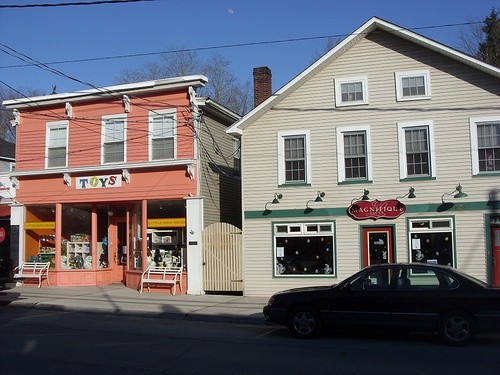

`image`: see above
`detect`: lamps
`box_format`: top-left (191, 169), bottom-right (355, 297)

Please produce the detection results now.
top-left (454, 182), bottom-right (467, 198)
top-left (159, 202), bottom-right (163, 210)
top-left (363, 189), bottom-right (369, 200)
top-left (51, 207), bottom-right (55, 213)
top-left (407, 186), bottom-right (417, 198)
top-left (272, 192), bottom-right (283, 204)
top-left (315, 191), bottom-right (326, 202)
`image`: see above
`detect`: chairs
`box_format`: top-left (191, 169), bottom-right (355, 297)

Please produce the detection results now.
top-left (401, 271), bottom-right (411, 288)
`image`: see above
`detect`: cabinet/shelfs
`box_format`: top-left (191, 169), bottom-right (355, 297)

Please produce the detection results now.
top-left (147, 228), bottom-right (185, 267)
top-left (67, 241), bottom-right (104, 269)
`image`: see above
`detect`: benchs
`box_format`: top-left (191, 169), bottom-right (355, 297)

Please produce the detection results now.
top-left (139, 266), bottom-right (183, 296)
top-left (12, 262), bottom-right (51, 288)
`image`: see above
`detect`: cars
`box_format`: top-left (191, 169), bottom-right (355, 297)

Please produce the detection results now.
top-left (263, 263), bottom-right (500, 339)
